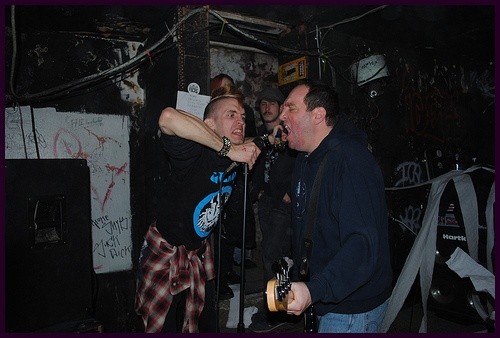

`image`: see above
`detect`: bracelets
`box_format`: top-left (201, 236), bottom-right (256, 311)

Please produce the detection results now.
top-left (263, 133), bottom-right (273, 149)
top-left (217, 136), bottom-right (231, 157)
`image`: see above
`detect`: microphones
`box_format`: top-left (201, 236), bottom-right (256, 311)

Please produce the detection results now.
top-left (222, 137), bottom-right (263, 180)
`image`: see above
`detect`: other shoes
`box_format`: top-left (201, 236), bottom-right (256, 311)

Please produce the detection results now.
top-left (218, 285), bottom-right (234, 300)
top-left (248, 309), bottom-right (289, 332)
top-left (221, 265), bottom-right (242, 285)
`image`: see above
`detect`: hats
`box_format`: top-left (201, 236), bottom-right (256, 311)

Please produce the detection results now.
top-left (258, 86), bottom-right (285, 103)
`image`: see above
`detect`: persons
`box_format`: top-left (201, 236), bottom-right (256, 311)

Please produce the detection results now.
top-left (134, 94), bottom-right (287, 332)
top-left (211, 73), bottom-right (303, 299)
top-left (279, 79), bottom-right (391, 332)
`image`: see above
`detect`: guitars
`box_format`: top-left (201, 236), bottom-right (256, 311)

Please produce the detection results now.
top-left (266, 266), bottom-right (319, 333)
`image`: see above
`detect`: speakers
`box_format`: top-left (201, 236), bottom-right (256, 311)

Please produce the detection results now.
top-left (5, 159), bottom-right (93, 333)
